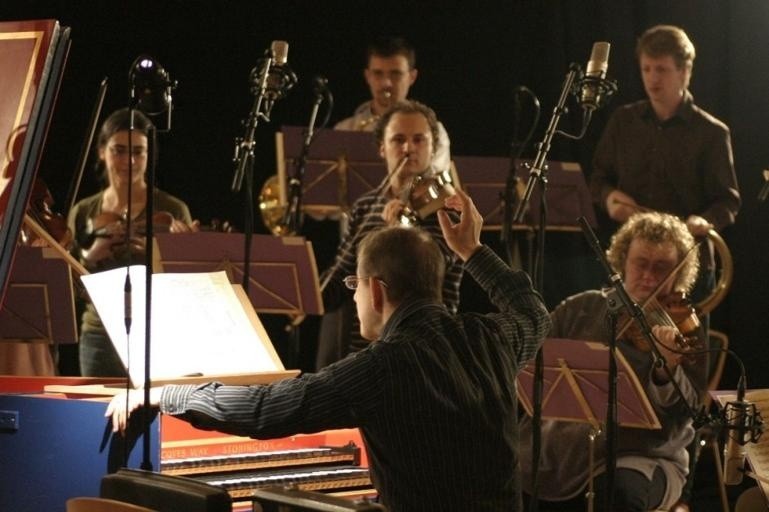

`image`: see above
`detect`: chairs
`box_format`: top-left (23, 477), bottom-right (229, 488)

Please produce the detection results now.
top-left (674, 326), bottom-right (731, 512)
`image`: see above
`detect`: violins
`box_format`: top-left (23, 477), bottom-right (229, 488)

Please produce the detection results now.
top-left (395, 171), bottom-right (452, 232)
top-left (85, 214), bottom-right (230, 255)
top-left (18, 176), bottom-right (92, 303)
top-left (620, 294), bottom-right (704, 366)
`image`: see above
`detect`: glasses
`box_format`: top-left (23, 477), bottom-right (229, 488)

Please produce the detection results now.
top-left (344, 275), bottom-right (390, 291)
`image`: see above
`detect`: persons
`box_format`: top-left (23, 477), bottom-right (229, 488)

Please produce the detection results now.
top-left (588, 25), bottom-right (742, 312)
top-left (68, 109), bottom-right (191, 377)
top-left (333, 38), bottom-right (452, 179)
top-left (523, 213), bottom-right (704, 509)
top-left (105, 178), bottom-right (552, 511)
top-left (289, 101), bottom-right (470, 354)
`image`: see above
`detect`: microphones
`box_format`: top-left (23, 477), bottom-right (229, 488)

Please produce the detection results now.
top-left (263, 40), bottom-right (288, 115)
top-left (725, 400), bottom-right (752, 485)
top-left (580, 41), bottom-right (611, 107)
